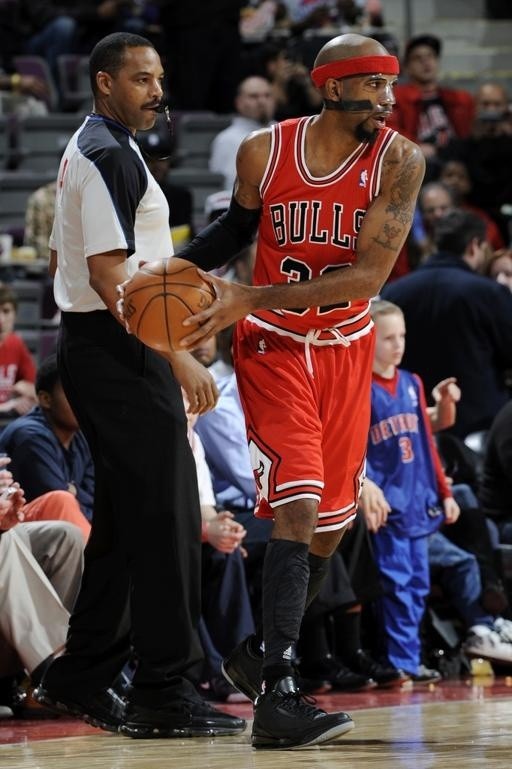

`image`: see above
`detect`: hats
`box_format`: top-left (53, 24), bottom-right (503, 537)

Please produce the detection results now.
top-left (402, 32), bottom-right (444, 66)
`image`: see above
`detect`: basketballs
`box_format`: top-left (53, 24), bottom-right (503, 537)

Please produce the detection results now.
top-left (121, 256), bottom-right (217, 351)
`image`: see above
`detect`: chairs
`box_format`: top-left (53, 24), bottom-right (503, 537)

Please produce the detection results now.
top-left (1, 45), bottom-right (251, 393)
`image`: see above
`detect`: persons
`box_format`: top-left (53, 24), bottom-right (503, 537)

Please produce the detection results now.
top-left (0, 1), bottom-right (512, 716)
top-left (116, 34), bottom-right (426, 749)
top-left (49, 32), bottom-right (248, 738)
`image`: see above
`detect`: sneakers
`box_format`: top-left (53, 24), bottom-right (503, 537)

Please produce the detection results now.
top-left (402, 663), bottom-right (443, 684)
top-left (247, 689), bottom-right (356, 751)
top-left (114, 688), bottom-right (247, 740)
top-left (466, 614), bottom-right (511, 666)
top-left (295, 663), bottom-right (332, 692)
top-left (342, 649), bottom-right (405, 685)
top-left (31, 652), bottom-right (127, 734)
top-left (319, 653), bottom-right (380, 693)
top-left (220, 633), bottom-right (267, 703)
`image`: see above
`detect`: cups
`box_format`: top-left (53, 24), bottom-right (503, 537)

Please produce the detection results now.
top-left (2, 234), bottom-right (12, 262)
top-left (1, 453), bottom-right (10, 495)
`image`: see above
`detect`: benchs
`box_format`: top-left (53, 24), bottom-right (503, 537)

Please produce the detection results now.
top-left (368, 1), bottom-right (512, 110)
